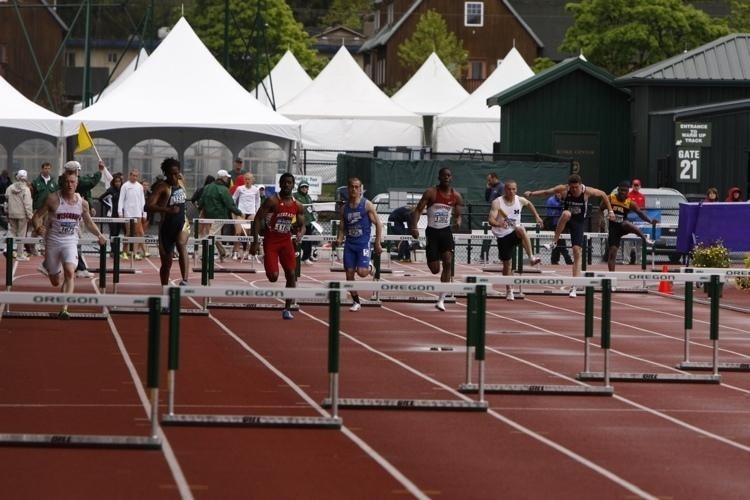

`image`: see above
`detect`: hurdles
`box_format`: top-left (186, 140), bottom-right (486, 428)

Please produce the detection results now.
top-left (70, 217), bottom-right (700, 274)
top-left (0, 291), bottom-right (170, 447)
top-left (374, 235), bottom-right (456, 302)
top-left (110, 235), bottom-right (213, 316)
top-left (291, 235), bottom-right (386, 307)
top-left (318, 280), bottom-right (493, 411)
top-left (204, 234), bottom-right (299, 311)
top-left (575, 270), bottom-right (725, 383)
top-left (453, 233), bottom-right (527, 299)
top-left (457, 275), bottom-right (615, 396)
top-left (1, 236), bottom-right (112, 318)
top-left (583, 231), bottom-right (649, 293)
top-left (511, 232), bottom-right (587, 295)
top-left (160, 285), bottom-right (346, 427)
top-left (675, 266), bottom-right (749, 371)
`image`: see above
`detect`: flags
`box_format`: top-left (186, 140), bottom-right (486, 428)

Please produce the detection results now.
top-left (72, 123), bottom-right (92, 155)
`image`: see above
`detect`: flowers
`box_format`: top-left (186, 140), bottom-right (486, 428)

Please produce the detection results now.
top-left (692, 238), bottom-right (731, 268)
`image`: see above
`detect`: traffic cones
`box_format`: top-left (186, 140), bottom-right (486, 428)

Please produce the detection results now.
top-left (656, 263), bottom-right (675, 295)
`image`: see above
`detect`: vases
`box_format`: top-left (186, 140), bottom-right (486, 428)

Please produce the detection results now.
top-left (704, 282), bottom-right (724, 298)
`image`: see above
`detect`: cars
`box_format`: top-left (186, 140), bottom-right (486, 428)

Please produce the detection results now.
top-left (370, 193), bottom-right (428, 247)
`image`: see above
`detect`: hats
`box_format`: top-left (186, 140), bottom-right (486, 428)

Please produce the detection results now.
top-left (17, 169), bottom-right (28, 179)
top-left (218, 169), bottom-right (232, 179)
top-left (65, 161), bottom-right (82, 170)
top-left (633, 179), bottom-right (641, 186)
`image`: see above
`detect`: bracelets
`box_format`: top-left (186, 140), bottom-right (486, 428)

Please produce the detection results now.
top-left (608, 211), bottom-right (613, 213)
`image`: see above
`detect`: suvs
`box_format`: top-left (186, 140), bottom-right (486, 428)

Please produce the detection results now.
top-left (596, 182), bottom-right (692, 263)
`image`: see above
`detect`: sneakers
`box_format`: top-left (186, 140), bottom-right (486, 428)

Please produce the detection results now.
top-left (569, 286), bottom-right (578, 298)
top-left (349, 301), bottom-right (362, 313)
top-left (434, 298), bottom-right (446, 311)
top-left (36, 263), bottom-right (49, 276)
top-left (504, 288), bottom-right (514, 301)
top-left (641, 238), bottom-right (657, 247)
top-left (543, 241), bottom-right (558, 251)
top-left (57, 309), bottom-right (72, 319)
top-left (529, 255), bottom-right (541, 267)
top-left (282, 309), bottom-right (294, 320)
top-left (16, 254), bottom-right (32, 262)
top-left (76, 270), bottom-right (97, 279)
top-left (111, 250), bottom-right (319, 264)
top-left (368, 263), bottom-right (377, 276)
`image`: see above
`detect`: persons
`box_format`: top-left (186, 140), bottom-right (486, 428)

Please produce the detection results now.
top-left (546, 184), bottom-right (574, 264)
top-left (723, 187), bottom-right (744, 201)
top-left (1, 155), bottom-right (384, 319)
top-left (484, 172), bottom-right (505, 206)
top-left (387, 205), bottom-right (423, 262)
top-left (522, 173), bottom-right (617, 298)
top-left (629, 179), bottom-right (645, 209)
top-left (599, 180), bottom-right (661, 270)
top-left (410, 168), bottom-right (463, 312)
top-left (487, 179), bottom-right (544, 300)
top-left (702, 186), bottom-right (720, 202)
